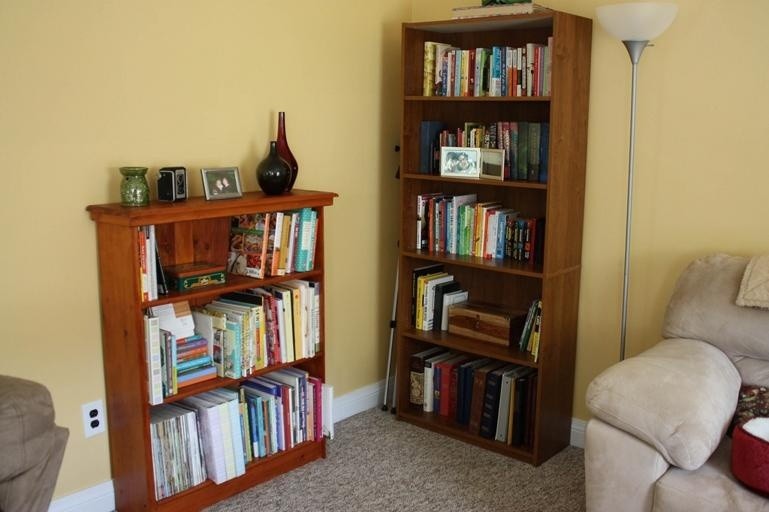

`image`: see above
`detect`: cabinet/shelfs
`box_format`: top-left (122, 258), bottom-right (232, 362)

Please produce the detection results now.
top-left (390, 11), bottom-right (595, 468)
top-left (84, 187), bottom-right (340, 511)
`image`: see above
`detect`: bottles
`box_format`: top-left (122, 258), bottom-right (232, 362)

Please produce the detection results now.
top-left (119, 167), bottom-right (151, 206)
top-left (254, 111), bottom-right (298, 195)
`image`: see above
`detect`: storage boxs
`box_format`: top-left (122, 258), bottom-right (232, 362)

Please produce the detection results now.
top-left (446, 297), bottom-right (527, 349)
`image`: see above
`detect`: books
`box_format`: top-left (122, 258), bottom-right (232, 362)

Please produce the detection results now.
top-left (227, 205), bottom-right (320, 279)
top-left (136, 223), bottom-right (226, 303)
top-left (415, 195), bottom-right (545, 267)
top-left (411, 264), bottom-right (543, 364)
top-left (145, 282), bottom-right (336, 501)
top-left (419, 120), bottom-right (548, 184)
top-left (410, 348), bottom-right (534, 445)
top-left (423, 36), bottom-right (554, 97)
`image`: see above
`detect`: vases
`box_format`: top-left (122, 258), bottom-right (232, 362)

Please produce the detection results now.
top-left (256, 111), bottom-right (299, 196)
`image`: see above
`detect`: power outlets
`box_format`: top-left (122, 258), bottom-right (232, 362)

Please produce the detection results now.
top-left (81, 399), bottom-right (108, 439)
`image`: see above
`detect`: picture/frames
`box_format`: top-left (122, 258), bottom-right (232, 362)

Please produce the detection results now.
top-left (439, 146), bottom-right (506, 182)
top-left (199, 166), bottom-right (242, 201)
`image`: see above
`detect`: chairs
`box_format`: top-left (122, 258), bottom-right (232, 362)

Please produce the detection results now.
top-left (583, 253), bottom-right (766, 510)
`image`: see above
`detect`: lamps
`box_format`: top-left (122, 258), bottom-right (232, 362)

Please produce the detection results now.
top-left (595, 5), bottom-right (680, 364)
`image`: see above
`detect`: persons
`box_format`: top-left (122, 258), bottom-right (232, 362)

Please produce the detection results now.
top-left (213, 178), bottom-right (224, 196)
top-left (222, 176), bottom-right (234, 194)
